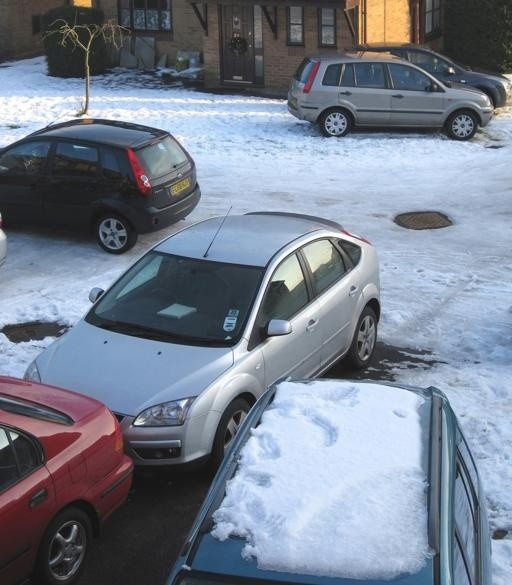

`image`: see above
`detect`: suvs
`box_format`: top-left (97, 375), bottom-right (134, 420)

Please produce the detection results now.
top-left (358, 42), bottom-right (509, 107)
top-left (291, 55), bottom-right (493, 142)
top-left (1, 117), bottom-right (202, 256)
top-left (165, 375), bottom-right (491, 585)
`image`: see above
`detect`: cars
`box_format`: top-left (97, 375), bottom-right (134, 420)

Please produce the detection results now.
top-left (0, 374), bottom-right (126, 585)
top-left (23, 206), bottom-right (381, 466)
